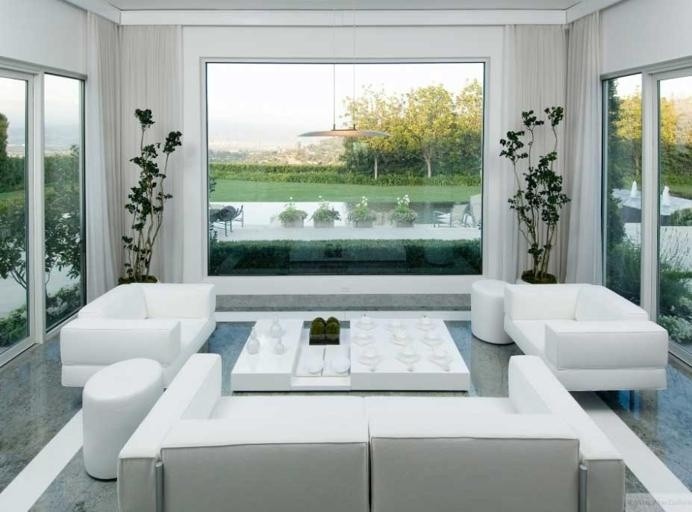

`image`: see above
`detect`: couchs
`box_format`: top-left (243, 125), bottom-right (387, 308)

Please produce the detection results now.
top-left (501, 281), bottom-right (669, 408)
top-left (116, 351), bottom-right (630, 511)
top-left (58, 282), bottom-right (221, 388)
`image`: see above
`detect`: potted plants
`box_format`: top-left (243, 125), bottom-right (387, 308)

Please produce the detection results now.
top-left (500, 105), bottom-right (573, 282)
top-left (117, 107), bottom-right (186, 285)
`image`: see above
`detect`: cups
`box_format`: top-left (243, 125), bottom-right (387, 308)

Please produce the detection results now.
top-left (351, 311), bottom-right (456, 372)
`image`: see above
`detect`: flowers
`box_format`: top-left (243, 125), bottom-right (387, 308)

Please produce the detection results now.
top-left (347, 196), bottom-right (377, 221)
top-left (278, 196), bottom-right (306, 220)
top-left (390, 194), bottom-right (419, 223)
top-left (313, 195), bottom-right (340, 222)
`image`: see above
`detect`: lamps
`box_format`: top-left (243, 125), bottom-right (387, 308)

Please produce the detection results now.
top-left (299, 9), bottom-right (390, 137)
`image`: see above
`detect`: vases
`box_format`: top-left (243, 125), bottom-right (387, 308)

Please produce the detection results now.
top-left (282, 219), bottom-right (304, 226)
top-left (396, 219), bottom-right (415, 227)
top-left (354, 219), bottom-right (373, 229)
top-left (314, 220), bottom-right (334, 228)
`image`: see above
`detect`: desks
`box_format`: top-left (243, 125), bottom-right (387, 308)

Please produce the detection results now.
top-left (79, 361), bottom-right (168, 482)
top-left (471, 279), bottom-right (519, 346)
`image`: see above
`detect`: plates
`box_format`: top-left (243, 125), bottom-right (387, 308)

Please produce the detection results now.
top-left (304, 354), bottom-right (324, 374)
top-left (331, 356), bottom-right (350, 373)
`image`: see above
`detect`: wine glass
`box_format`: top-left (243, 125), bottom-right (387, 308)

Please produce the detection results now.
top-left (245, 328), bottom-right (260, 354)
top-left (272, 316), bottom-right (281, 337)
top-left (275, 331), bottom-right (284, 355)
